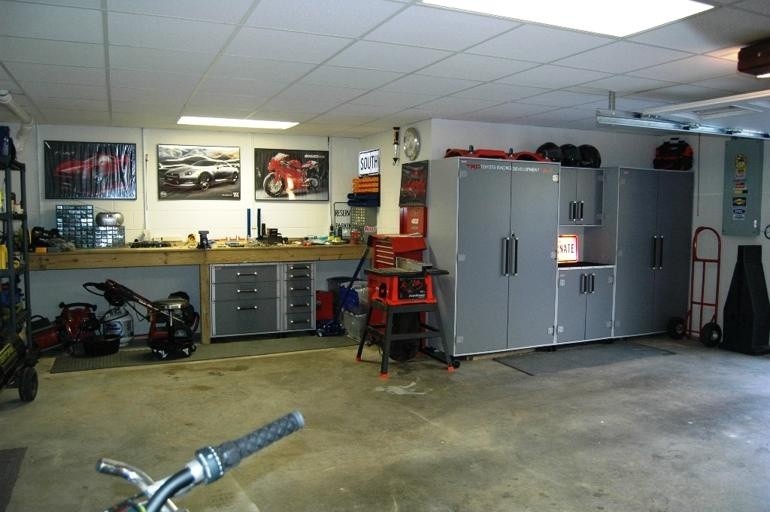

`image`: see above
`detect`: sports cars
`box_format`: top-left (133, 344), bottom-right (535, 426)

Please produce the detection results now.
top-left (54, 154), bottom-right (129, 185)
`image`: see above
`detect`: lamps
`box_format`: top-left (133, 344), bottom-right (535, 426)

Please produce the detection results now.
top-left (737, 38), bottom-right (769, 79)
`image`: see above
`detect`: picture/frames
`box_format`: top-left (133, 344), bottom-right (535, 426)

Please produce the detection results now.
top-left (358, 148), bottom-right (380, 178)
top-left (44, 140), bottom-right (330, 201)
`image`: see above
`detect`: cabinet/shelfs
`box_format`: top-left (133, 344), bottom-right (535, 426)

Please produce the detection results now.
top-left (209, 261), bottom-right (317, 339)
top-left (558, 167), bottom-right (604, 226)
top-left (1, 158), bottom-right (38, 402)
top-left (427, 160), bottom-right (557, 357)
top-left (557, 260), bottom-right (615, 346)
top-left (585, 167), bottom-right (693, 346)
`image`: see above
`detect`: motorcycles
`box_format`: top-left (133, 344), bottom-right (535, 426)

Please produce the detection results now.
top-left (263, 153), bottom-right (319, 197)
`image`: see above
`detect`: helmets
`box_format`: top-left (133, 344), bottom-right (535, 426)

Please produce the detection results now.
top-left (537, 142), bottom-right (601, 168)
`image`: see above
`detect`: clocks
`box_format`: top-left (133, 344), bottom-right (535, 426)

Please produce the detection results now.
top-left (403, 128), bottom-right (420, 161)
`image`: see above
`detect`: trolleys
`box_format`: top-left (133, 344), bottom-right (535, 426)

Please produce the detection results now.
top-left (666, 225), bottom-right (724, 349)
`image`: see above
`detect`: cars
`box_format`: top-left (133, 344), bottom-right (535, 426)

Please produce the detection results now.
top-left (164, 159), bottom-right (238, 191)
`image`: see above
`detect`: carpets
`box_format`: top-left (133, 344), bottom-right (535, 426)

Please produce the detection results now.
top-left (0, 446), bottom-right (29, 512)
top-left (493, 341), bottom-right (676, 376)
top-left (49, 334), bottom-right (360, 374)
